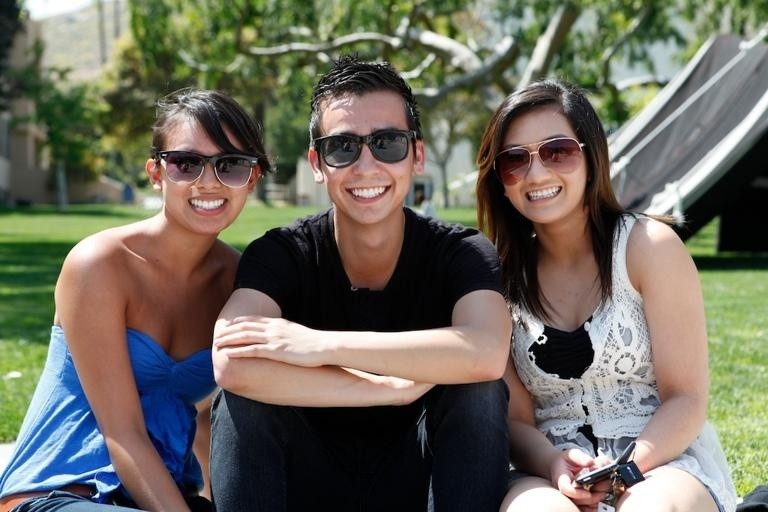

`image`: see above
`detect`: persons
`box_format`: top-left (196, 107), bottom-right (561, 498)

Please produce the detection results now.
top-left (0, 87), bottom-right (275, 511)
top-left (474, 79), bottom-right (738, 512)
top-left (208, 51), bottom-right (514, 512)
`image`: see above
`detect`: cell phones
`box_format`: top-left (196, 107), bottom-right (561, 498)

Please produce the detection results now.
top-left (575, 463), bottom-right (617, 485)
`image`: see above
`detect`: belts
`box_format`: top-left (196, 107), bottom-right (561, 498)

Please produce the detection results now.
top-left (0, 484), bottom-right (96, 512)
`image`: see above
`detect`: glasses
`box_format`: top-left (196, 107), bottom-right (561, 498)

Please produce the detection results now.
top-left (493, 135), bottom-right (586, 186)
top-left (154, 150), bottom-right (259, 189)
top-left (310, 129), bottom-right (416, 169)
